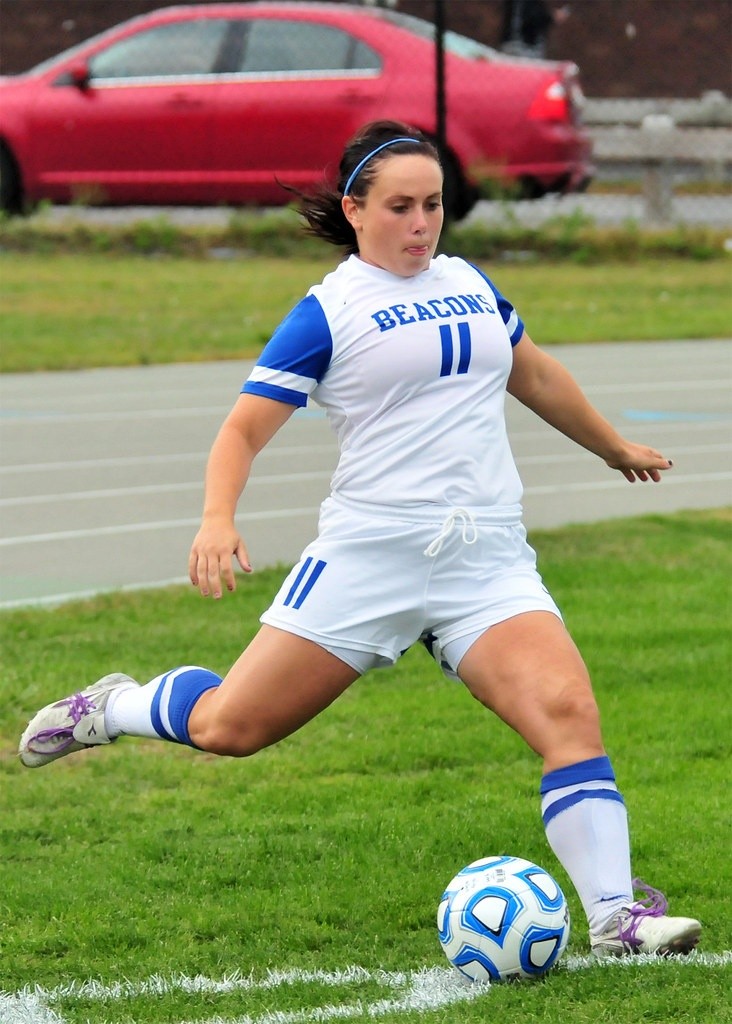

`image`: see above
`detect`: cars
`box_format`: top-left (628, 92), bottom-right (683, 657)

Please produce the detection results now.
top-left (0, 1), bottom-right (596, 223)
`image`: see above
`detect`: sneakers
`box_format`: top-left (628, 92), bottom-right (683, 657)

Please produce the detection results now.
top-left (19, 671), bottom-right (141, 769)
top-left (587, 901), bottom-right (702, 957)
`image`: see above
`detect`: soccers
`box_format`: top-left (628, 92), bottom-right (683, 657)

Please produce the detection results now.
top-left (437, 858), bottom-right (570, 985)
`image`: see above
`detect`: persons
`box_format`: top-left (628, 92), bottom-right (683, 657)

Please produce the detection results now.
top-left (20, 120), bottom-right (701, 955)
top-left (546, 0), bottom-right (588, 68)
